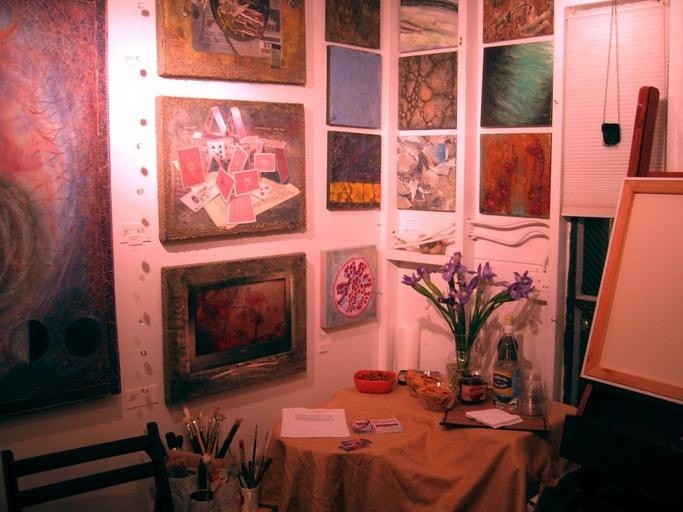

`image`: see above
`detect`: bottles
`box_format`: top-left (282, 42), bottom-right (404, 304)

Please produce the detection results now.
top-left (491, 324), bottom-right (521, 404)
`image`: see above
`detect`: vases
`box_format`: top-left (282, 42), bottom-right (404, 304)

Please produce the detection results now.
top-left (446, 331), bottom-right (486, 398)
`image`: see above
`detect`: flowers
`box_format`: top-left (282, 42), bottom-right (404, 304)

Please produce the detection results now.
top-left (401, 251), bottom-right (539, 386)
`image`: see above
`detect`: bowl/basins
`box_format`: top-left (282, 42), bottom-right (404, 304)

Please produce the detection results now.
top-left (415, 381), bottom-right (460, 411)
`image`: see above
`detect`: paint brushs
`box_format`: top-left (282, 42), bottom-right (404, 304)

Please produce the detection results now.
top-left (165, 403), bottom-right (273, 502)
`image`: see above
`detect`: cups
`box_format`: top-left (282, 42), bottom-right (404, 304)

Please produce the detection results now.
top-left (149, 465), bottom-right (198, 512)
top-left (188, 489), bottom-right (215, 512)
top-left (238, 484), bottom-right (260, 511)
top-left (520, 368), bottom-right (545, 418)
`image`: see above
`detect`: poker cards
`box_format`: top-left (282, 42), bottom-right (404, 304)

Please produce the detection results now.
top-left (172, 107), bottom-right (290, 224)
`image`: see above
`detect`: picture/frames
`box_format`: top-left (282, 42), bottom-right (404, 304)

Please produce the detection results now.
top-left (161, 251), bottom-right (308, 406)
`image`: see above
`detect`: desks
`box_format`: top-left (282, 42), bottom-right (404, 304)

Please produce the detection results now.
top-left (271, 386), bottom-right (577, 511)
